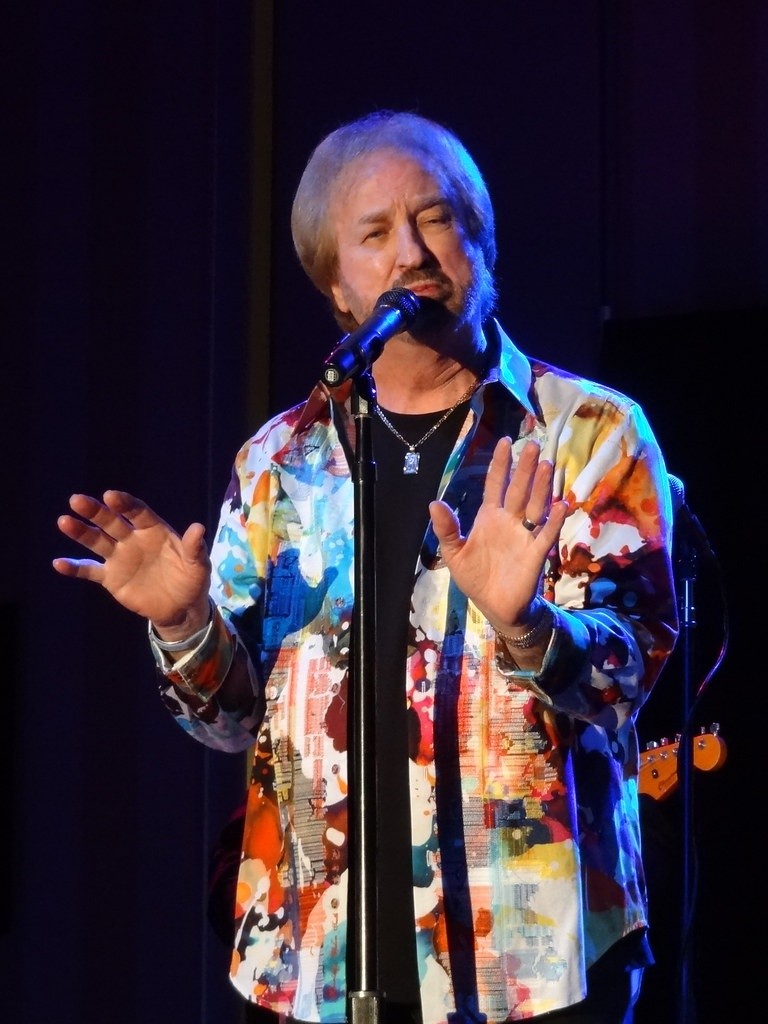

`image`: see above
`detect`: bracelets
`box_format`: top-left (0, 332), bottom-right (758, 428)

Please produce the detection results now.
top-left (490, 610), bottom-right (550, 650)
top-left (151, 624), bottom-right (210, 652)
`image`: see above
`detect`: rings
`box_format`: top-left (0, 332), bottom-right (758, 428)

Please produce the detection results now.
top-left (523, 517), bottom-right (538, 531)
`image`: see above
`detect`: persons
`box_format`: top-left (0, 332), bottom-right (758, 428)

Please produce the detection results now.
top-left (51, 110), bottom-right (680, 1024)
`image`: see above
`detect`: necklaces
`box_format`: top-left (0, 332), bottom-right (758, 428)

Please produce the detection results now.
top-left (375, 377), bottom-right (481, 475)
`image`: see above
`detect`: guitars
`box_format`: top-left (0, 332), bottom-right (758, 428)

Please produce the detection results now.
top-left (634, 718), bottom-right (731, 804)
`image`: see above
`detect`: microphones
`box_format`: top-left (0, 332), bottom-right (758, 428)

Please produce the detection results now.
top-left (665, 472), bottom-right (714, 561)
top-left (316, 287), bottom-right (421, 388)
